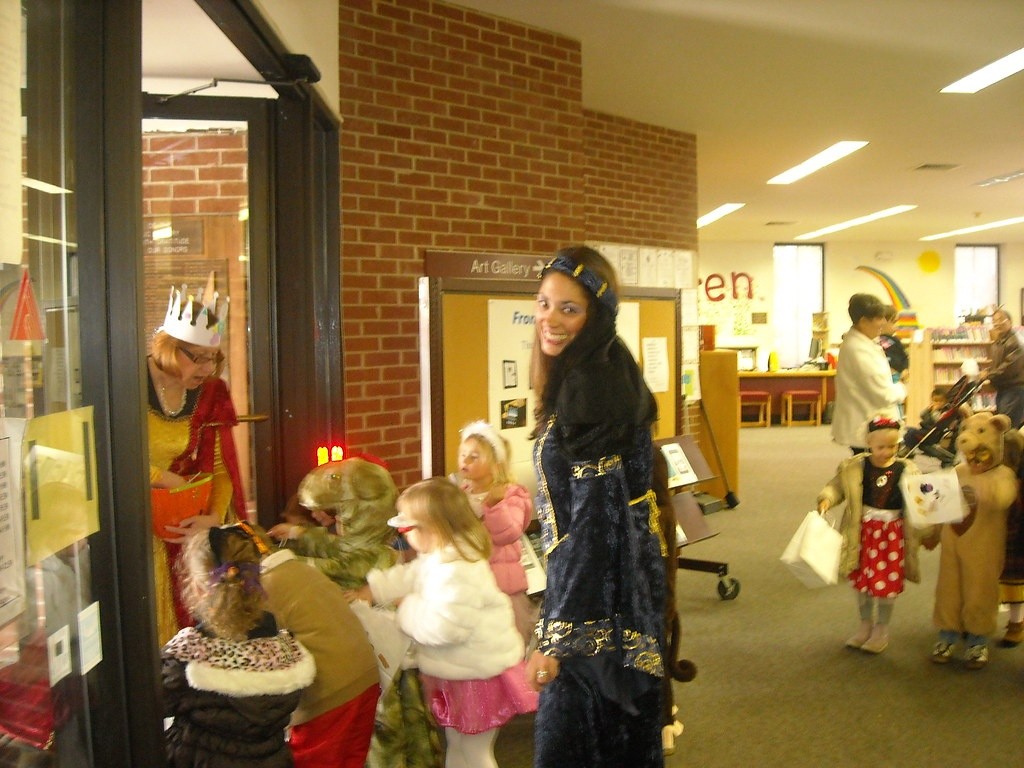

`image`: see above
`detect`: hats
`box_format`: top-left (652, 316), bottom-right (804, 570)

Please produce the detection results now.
top-left (387, 495), bottom-right (467, 526)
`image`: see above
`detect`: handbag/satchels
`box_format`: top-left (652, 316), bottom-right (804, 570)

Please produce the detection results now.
top-left (781, 510), bottom-right (842, 589)
top-left (900, 467), bottom-right (970, 528)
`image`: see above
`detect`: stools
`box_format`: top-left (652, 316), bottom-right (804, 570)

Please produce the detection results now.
top-left (738, 390), bottom-right (771, 427)
top-left (780, 389), bottom-right (822, 428)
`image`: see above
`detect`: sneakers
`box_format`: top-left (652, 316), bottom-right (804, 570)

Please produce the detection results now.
top-left (932, 641), bottom-right (956, 661)
top-left (965, 645), bottom-right (989, 669)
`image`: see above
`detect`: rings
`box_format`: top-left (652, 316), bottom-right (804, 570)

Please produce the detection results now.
top-left (536, 671), bottom-right (549, 678)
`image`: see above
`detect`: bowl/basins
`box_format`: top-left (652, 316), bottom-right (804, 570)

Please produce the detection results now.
top-left (150, 472), bottom-right (214, 538)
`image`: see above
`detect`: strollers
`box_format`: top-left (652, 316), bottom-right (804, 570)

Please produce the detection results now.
top-left (895, 374), bottom-right (991, 465)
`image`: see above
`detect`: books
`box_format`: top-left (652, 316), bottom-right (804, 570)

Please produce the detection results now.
top-left (931, 326), bottom-right (992, 383)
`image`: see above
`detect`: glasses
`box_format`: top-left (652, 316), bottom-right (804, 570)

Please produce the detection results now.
top-left (178, 346), bottom-right (224, 365)
top-left (395, 527), bottom-right (413, 538)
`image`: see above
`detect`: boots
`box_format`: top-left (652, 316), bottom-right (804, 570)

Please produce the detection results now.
top-left (860, 623), bottom-right (890, 652)
top-left (1002, 621), bottom-right (1024, 645)
top-left (848, 619), bottom-right (873, 648)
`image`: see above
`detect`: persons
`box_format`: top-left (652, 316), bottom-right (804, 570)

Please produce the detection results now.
top-left (924, 403), bottom-right (1020, 669)
top-left (343, 478), bottom-right (541, 768)
top-left (832, 293), bottom-right (911, 457)
top-left (144, 300), bottom-right (249, 645)
top-left (512, 245), bottom-right (678, 768)
top-left (159, 525), bottom-right (316, 768)
top-left (274, 457), bottom-right (443, 768)
top-left (818, 415), bottom-right (929, 653)
top-left (454, 421), bottom-right (533, 649)
top-left (983, 309), bottom-right (1024, 428)
top-left (7, 497), bottom-right (91, 647)
top-left (252, 526), bottom-right (380, 768)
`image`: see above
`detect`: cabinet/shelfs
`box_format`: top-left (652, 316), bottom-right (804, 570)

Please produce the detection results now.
top-left (905, 340), bottom-right (996, 426)
